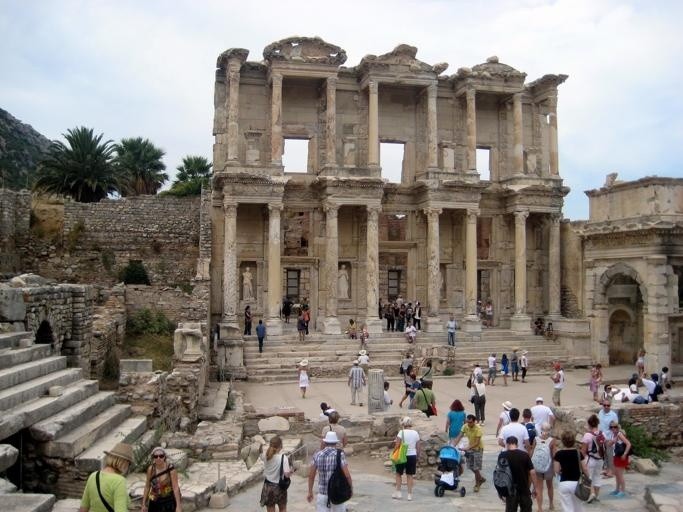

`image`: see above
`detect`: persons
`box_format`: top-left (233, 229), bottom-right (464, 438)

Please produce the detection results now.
top-left (379, 351), bottom-right (438, 417)
top-left (242, 267), bottom-right (253, 300)
top-left (445, 315), bottom-right (460, 346)
top-left (390, 399), bottom-right (487, 501)
top-left (548, 349), bottom-right (670, 406)
top-left (477, 300), bottom-right (495, 329)
top-left (308, 431), bottom-right (353, 512)
top-left (379, 297), bottom-right (422, 343)
top-left (283, 296), bottom-right (310, 341)
top-left (348, 360), bottom-right (366, 406)
top-left (359, 323), bottom-right (369, 350)
top-left (245, 306), bottom-right (251, 335)
top-left (256, 320), bottom-right (265, 353)
top-left (344, 319), bottom-right (357, 339)
top-left (78, 442), bottom-right (133, 512)
top-left (141, 447), bottom-right (182, 512)
top-left (358, 350), bottom-right (369, 376)
top-left (467, 348), bottom-right (528, 425)
top-left (320, 403), bottom-right (336, 422)
top-left (338, 264), bottom-right (350, 298)
top-left (534, 318), bottom-right (556, 339)
top-left (259, 436), bottom-right (294, 512)
top-left (494, 395), bottom-right (631, 512)
top-left (321, 411), bottom-right (347, 449)
top-left (299, 359), bottom-right (309, 398)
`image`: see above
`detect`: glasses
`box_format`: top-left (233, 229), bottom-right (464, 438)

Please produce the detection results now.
top-left (603, 404), bottom-right (610, 407)
top-left (609, 426), bottom-right (617, 429)
top-left (153, 454), bottom-right (164, 459)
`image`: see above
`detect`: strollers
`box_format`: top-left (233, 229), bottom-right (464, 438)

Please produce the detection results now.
top-left (433, 445), bottom-right (465, 497)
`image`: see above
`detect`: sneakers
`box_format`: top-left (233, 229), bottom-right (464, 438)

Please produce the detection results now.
top-left (587, 493), bottom-right (595, 503)
top-left (610, 490), bottom-right (624, 497)
top-left (474, 477), bottom-right (486, 491)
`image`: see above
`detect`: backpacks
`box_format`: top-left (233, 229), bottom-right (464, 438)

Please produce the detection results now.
top-left (531, 436), bottom-right (553, 473)
top-left (587, 431), bottom-right (606, 460)
top-left (653, 382), bottom-right (663, 396)
top-left (328, 450), bottom-right (351, 505)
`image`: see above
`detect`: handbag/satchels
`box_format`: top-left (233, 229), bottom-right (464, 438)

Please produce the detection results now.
top-left (400, 366), bottom-right (404, 374)
top-left (427, 405), bottom-right (434, 415)
top-left (279, 476), bottom-right (291, 489)
top-left (471, 394), bottom-right (479, 403)
top-left (467, 379), bottom-right (472, 388)
top-left (493, 449), bottom-right (518, 498)
top-left (390, 442), bottom-right (408, 464)
top-left (615, 442), bottom-right (633, 456)
top-left (575, 475), bottom-right (592, 501)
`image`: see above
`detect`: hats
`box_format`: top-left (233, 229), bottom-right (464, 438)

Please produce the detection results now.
top-left (502, 401), bottom-right (512, 411)
top-left (104, 444), bottom-right (134, 463)
top-left (359, 350), bottom-right (366, 355)
top-left (322, 431), bottom-right (341, 444)
top-left (300, 360), bottom-right (308, 367)
top-left (630, 384), bottom-right (638, 392)
top-left (241, 443), bottom-right (261, 470)
top-left (523, 349), bottom-right (528, 355)
